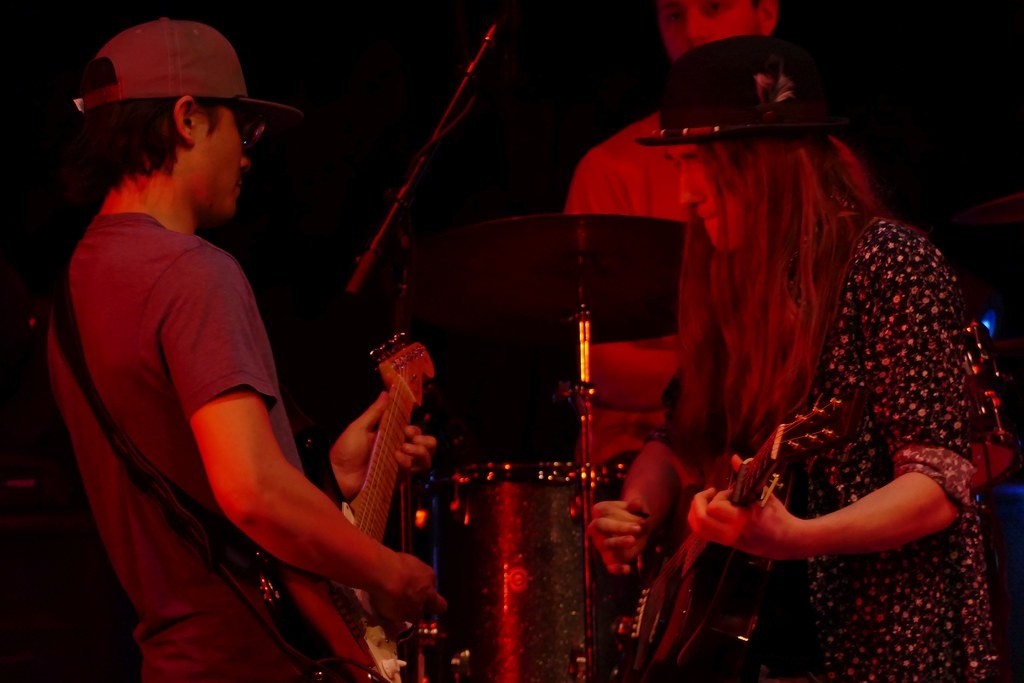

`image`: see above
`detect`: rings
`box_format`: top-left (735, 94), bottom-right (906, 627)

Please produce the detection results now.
top-left (424, 597), bottom-right (433, 608)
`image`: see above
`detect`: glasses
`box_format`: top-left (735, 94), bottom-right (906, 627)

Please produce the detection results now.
top-left (199, 98), bottom-right (266, 145)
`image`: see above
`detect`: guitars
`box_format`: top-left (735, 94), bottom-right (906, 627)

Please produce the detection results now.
top-left (259, 327), bottom-right (436, 682)
top-left (627, 385), bottom-right (877, 683)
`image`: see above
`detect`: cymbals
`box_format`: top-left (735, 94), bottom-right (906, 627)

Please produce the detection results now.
top-left (408, 206), bottom-right (686, 342)
top-left (949, 191), bottom-right (1024, 225)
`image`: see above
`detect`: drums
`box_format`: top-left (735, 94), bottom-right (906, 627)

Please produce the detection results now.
top-left (421, 457), bottom-right (629, 683)
top-left (968, 353), bottom-right (1023, 491)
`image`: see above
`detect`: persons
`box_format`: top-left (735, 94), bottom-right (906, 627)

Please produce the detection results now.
top-left (562, 0), bottom-right (1001, 683)
top-left (46, 20), bottom-right (450, 683)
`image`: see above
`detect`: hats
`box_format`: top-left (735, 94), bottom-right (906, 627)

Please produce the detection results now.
top-left (73, 17), bottom-right (303, 123)
top-left (636, 34), bottom-right (856, 147)
